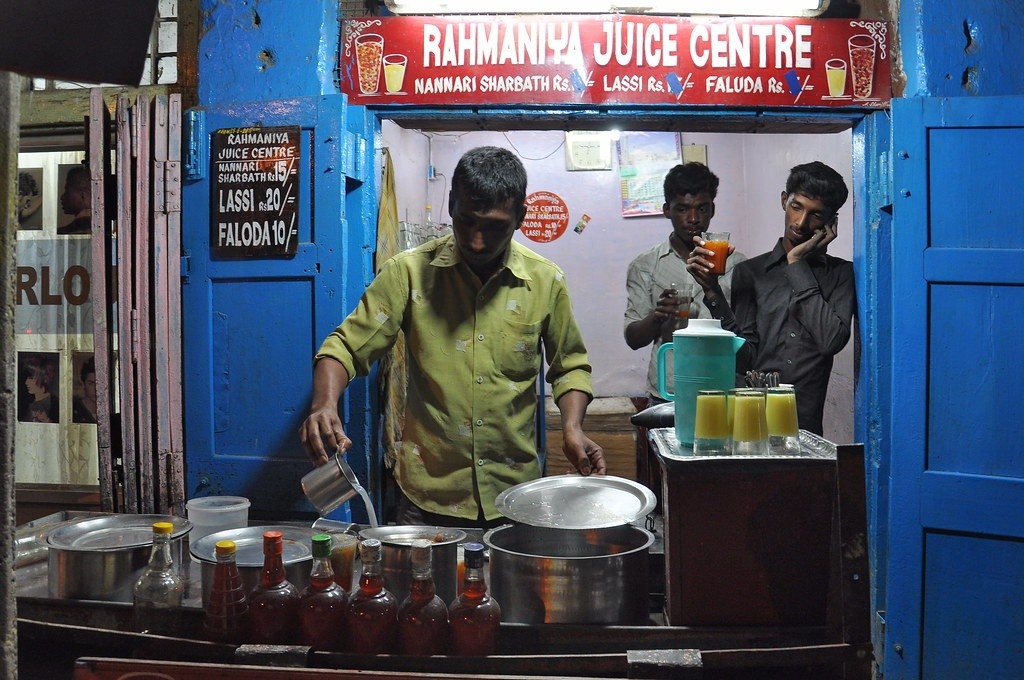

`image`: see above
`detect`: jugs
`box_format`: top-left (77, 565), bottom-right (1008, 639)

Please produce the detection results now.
top-left (656, 319), bottom-right (747, 448)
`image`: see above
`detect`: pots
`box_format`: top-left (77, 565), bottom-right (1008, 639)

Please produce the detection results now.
top-left (45, 515), bottom-right (189, 608)
top-left (358, 526), bottom-right (465, 610)
top-left (482, 523), bottom-right (657, 628)
top-left (190, 526), bottom-right (322, 607)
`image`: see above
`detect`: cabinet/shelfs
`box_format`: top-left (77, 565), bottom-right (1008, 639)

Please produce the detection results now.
top-left (629, 395), bottom-right (835, 628)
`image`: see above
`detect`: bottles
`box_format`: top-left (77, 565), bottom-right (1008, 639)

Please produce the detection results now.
top-left (203, 541), bottom-right (248, 646)
top-left (450, 542), bottom-right (500, 658)
top-left (349, 539), bottom-right (400, 654)
top-left (248, 530), bottom-right (300, 646)
top-left (132, 523), bottom-right (184, 639)
top-left (400, 538), bottom-right (450, 657)
top-left (300, 534), bottom-right (348, 651)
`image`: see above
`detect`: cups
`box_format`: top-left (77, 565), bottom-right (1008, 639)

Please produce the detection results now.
top-left (747, 384), bottom-right (795, 397)
top-left (13, 517), bottom-right (87, 569)
top-left (187, 498), bottom-right (251, 542)
top-left (693, 387), bottom-right (729, 457)
top-left (300, 445), bottom-right (361, 516)
top-left (764, 386), bottom-right (801, 457)
top-left (727, 388), bottom-right (754, 455)
top-left (729, 390), bottom-right (768, 456)
top-left (312, 515), bottom-right (362, 560)
top-left (700, 231), bottom-right (729, 275)
top-left (670, 282), bottom-right (693, 319)
top-left (325, 529), bottom-right (358, 599)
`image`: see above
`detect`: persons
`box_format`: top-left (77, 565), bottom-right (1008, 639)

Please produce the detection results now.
top-left (17, 166), bottom-right (91, 235)
top-left (623, 162), bottom-right (750, 409)
top-left (299, 146), bottom-right (606, 535)
top-left (72, 357), bottom-right (98, 424)
top-left (686, 161), bottom-right (856, 438)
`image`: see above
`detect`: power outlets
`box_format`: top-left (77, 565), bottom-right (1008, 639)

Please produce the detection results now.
top-left (428, 165), bottom-right (436, 180)
